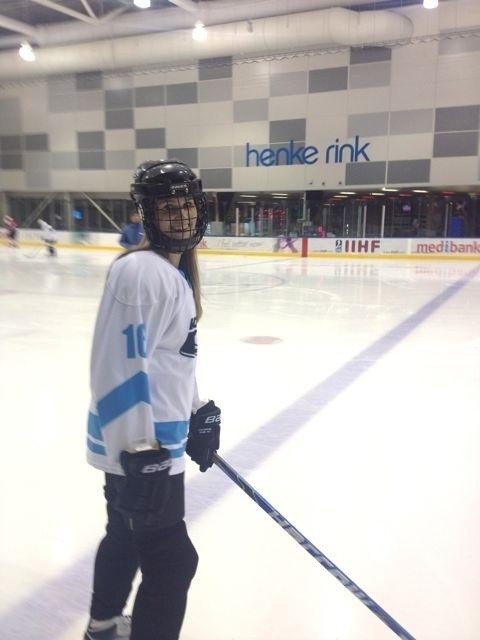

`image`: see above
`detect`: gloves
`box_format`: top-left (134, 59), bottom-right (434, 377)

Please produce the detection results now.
top-left (103, 446), bottom-right (172, 526)
top-left (185, 398), bottom-right (221, 472)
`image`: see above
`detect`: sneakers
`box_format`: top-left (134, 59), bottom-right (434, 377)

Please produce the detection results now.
top-left (84, 613), bottom-right (132, 639)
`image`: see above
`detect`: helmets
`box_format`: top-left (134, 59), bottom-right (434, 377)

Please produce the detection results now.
top-left (131, 158), bottom-right (208, 253)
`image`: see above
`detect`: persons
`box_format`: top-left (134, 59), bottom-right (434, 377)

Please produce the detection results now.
top-left (117, 209), bottom-right (148, 250)
top-left (83, 158), bottom-right (222, 640)
top-left (36, 218), bottom-right (59, 257)
top-left (3, 215), bottom-right (19, 248)
